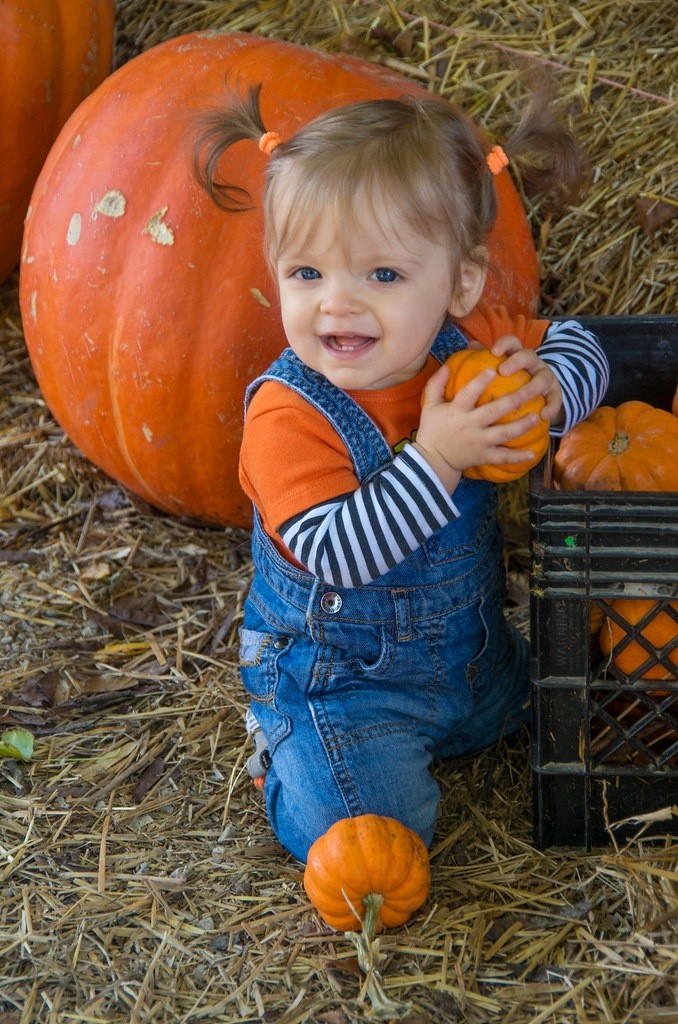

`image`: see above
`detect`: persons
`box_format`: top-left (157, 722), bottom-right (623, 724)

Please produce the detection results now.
top-left (185, 66), bottom-right (610, 861)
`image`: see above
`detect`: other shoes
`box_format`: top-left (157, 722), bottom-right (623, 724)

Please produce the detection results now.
top-left (245, 709), bottom-right (272, 780)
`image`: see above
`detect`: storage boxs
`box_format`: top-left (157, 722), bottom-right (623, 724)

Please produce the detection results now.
top-left (526, 313), bottom-right (678, 857)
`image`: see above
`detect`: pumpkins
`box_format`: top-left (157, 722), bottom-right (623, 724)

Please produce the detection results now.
top-left (0, 0), bottom-right (678, 939)
